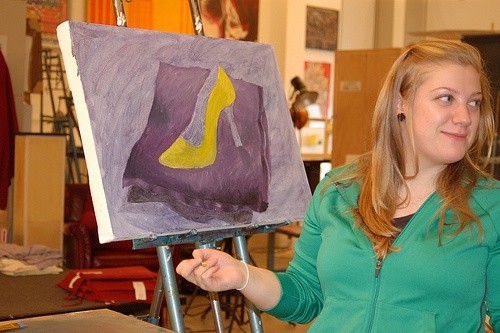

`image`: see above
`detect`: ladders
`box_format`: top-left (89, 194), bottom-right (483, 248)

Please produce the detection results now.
top-left (39, 52), bottom-right (88, 183)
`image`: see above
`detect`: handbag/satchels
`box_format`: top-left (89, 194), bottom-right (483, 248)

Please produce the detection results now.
top-left (55, 265), bottom-right (167, 307)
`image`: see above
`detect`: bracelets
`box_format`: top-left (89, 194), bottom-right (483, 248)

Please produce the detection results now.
top-left (236, 261), bottom-right (250, 290)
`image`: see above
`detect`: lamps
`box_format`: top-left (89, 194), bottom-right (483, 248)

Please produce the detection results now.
top-left (289, 76), bottom-right (319, 111)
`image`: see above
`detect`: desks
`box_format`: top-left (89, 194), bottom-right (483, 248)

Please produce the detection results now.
top-left (0, 309), bottom-right (177, 333)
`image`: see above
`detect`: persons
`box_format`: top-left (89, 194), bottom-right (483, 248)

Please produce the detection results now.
top-left (175, 39), bottom-right (500, 333)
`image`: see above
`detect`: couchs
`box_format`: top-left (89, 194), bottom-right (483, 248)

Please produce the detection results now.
top-left (63, 184), bottom-right (195, 292)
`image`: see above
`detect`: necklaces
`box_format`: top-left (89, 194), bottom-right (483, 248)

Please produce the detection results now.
top-left (399, 189), bottom-right (434, 208)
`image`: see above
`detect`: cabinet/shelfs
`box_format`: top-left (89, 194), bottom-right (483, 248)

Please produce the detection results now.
top-left (332, 48), bottom-right (401, 170)
